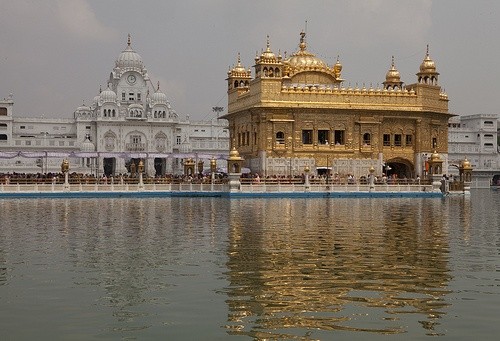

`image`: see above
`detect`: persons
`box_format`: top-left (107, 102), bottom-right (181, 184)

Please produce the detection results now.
top-left (0, 156), bottom-right (457, 192)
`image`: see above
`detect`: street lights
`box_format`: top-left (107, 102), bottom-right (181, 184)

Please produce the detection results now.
top-left (213, 105), bottom-right (224, 149)
